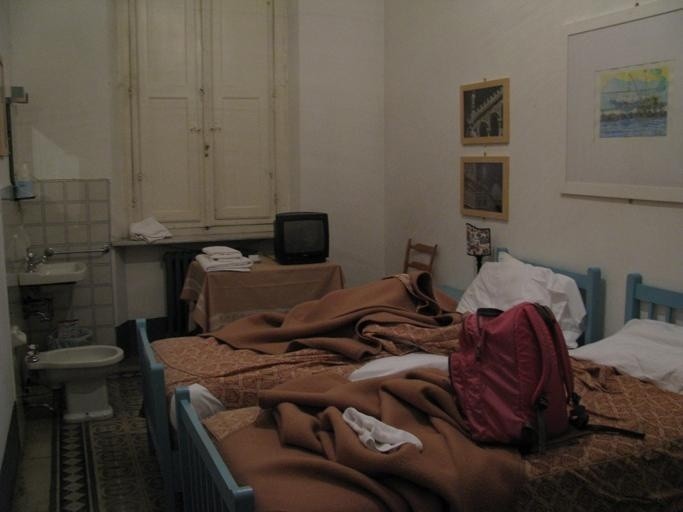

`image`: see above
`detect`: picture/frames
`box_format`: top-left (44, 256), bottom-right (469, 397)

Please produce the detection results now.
top-left (557, 1), bottom-right (682, 204)
top-left (457, 77), bottom-right (511, 147)
top-left (459, 152), bottom-right (510, 221)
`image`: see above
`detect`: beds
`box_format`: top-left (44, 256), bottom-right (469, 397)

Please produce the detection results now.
top-left (137, 246), bottom-right (600, 511)
top-left (175, 272), bottom-right (682, 511)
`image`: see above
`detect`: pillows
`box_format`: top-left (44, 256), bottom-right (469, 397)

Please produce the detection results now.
top-left (495, 252), bottom-right (588, 350)
top-left (457, 262), bottom-right (567, 329)
top-left (568, 316), bottom-right (682, 395)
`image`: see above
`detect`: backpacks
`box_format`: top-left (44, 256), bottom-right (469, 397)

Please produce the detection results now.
top-left (448, 302), bottom-right (590, 456)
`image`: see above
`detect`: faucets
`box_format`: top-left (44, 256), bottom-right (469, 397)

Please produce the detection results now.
top-left (26, 347), bottom-right (41, 362)
top-left (28, 252), bottom-right (46, 271)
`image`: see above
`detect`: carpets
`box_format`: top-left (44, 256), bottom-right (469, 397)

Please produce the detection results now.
top-left (50, 370), bottom-right (181, 512)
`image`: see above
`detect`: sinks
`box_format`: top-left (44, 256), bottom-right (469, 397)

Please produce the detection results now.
top-left (23, 344), bottom-right (124, 389)
top-left (18, 263), bottom-right (86, 287)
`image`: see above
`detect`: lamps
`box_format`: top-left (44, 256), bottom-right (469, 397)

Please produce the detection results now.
top-left (466, 222), bottom-right (491, 269)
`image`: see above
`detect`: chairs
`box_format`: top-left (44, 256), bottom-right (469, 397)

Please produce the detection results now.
top-left (402, 236), bottom-right (439, 277)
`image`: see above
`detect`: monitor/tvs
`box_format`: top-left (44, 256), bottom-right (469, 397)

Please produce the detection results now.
top-left (274, 212), bottom-right (329, 264)
